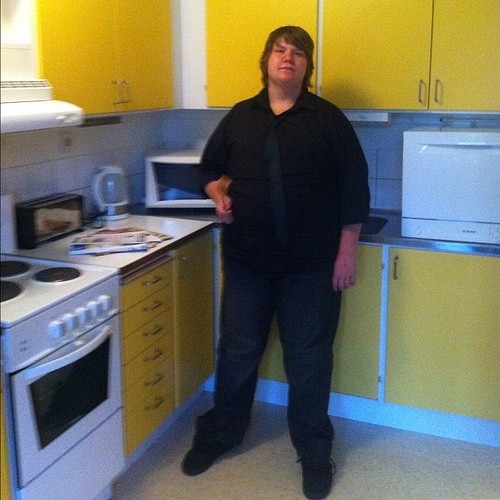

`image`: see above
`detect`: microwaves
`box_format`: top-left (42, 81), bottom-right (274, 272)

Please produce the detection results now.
top-left (145, 148), bottom-right (217, 211)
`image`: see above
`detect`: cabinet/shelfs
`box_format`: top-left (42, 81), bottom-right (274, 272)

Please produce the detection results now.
top-left (384, 248), bottom-right (500, 423)
top-left (36, 0), bottom-right (175, 118)
top-left (204, 0), bottom-right (319, 109)
top-left (319, 0), bottom-right (499, 114)
top-left (120, 232), bottom-right (215, 459)
top-left (220, 229), bottom-right (384, 402)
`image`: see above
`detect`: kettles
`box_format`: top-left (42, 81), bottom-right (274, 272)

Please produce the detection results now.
top-left (92, 166), bottom-right (130, 221)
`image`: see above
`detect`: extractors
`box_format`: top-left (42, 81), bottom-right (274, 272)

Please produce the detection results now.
top-left (1, 80), bottom-right (83, 135)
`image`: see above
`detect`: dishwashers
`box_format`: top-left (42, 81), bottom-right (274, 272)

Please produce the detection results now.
top-left (1, 313), bottom-right (125, 499)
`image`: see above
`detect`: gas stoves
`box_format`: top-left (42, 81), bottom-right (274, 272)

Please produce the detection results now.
top-left (1, 253), bottom-right (120, 374)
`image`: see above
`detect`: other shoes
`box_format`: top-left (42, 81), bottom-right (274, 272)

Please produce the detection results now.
top-left (181, 434), bottom-right (243, 475)
top-left (296, 455), bottom-right (336, 500)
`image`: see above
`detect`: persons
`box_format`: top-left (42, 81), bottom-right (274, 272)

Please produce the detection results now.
top-left (180, 26), bottom-right (370, 499)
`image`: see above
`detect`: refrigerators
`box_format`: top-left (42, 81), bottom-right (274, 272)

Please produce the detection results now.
top-left (401, 124), bottom-right (500, 245)
